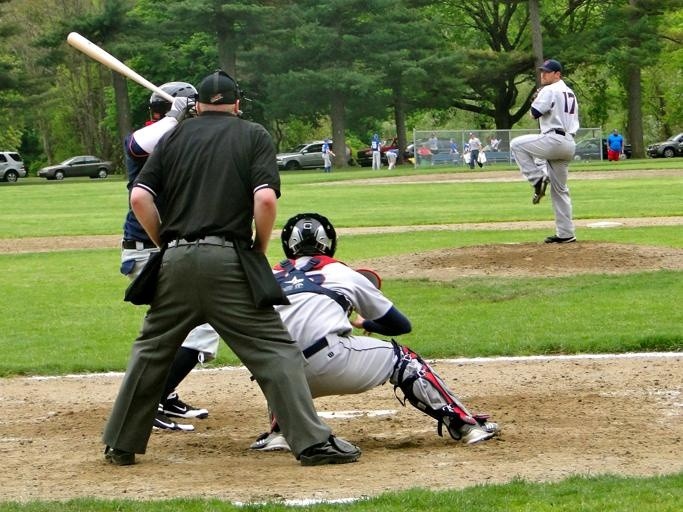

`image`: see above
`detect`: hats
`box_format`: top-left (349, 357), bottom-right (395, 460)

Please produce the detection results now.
top-left (537, 60), bottom-right (561, 72)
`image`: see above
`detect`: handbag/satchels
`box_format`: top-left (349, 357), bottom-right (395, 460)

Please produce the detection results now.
top-left (464, 152), bottom-right (470, 163)
top-left (478, 153), bottom-right (486, 163)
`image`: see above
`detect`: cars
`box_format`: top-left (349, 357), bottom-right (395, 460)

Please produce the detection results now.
top-left (570, 138), bottom-right (633, 162)
top-left (36, 154), bottom-right (117, 180)
top-left (647, 133), bottom-right (683, 157)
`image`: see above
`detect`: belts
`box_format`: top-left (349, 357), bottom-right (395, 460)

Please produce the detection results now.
top-left (555, 129), bottom-right (564, 135)
top-left (167, 236), bottom-right (233, 248)
top-left (303, 338), bottom-right (327, 358)
top-left (122, 241), bottom-right (156, 249)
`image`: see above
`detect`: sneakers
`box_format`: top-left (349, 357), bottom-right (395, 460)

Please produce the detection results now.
top-left (533, 176), bottom-right (550, 204)
top-left (158, 392), bottom-right (208, 418)
top-left (299, 436), bottom-right (361, 466)
top-left (461, 420), bottom-right (495, 444)
top-left (544, 235), bottom-right (576, 243)
top-left (152, 403), bottom-right (194, 432)
top-left (105, 445), bottom-right (134, 465)
top-left (249, 432), bottom-right (290, 452)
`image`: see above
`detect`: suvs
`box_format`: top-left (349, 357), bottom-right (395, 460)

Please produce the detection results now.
top-left (0, 151), bottom-right (26, 184)
top-left (403, 137), bottom-right (456, 162)
top-left (273, 139), bottom-right (354, 172)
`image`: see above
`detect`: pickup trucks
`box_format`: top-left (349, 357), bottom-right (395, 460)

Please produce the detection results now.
top-left (356, 136), bottom-right (401, 167)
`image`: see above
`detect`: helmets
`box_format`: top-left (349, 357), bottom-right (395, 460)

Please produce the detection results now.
top-left (198, 72), bottom-right (254, 121)
top-left (149, 82), bottom-right (198, 119)
top-left (282, 213), bottom-right (335, 259)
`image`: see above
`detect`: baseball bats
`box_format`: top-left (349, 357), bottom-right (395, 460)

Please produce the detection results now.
top-left (66, 31), bottom-right (195, 115)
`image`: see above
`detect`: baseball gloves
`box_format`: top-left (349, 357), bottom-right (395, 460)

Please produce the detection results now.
top-left (530, 86), bottom-right (542, 100)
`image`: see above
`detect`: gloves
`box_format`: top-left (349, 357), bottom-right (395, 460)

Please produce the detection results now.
top-left (165, 97), bottom-right (196, 122)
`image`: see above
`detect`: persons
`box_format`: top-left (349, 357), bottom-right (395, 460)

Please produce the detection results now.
top-left (249, 212), bottom-right (501, 452)
top-left (100, 70), bottom-right (361, 467)
top-left (371, 134), bottom-right (385, 170)
top-left (386, 149), bottom-right (399, 170)
top-left (321, 138), bottom-right (336, 172)
top-left (417, 132), bottom-right (499, 170)
top-left (607, 128), bottom-right (624, 161)
top-left (508, 59), bottom-right (579, 244)
top-left (119, 81), bottom-right (221, 433)
